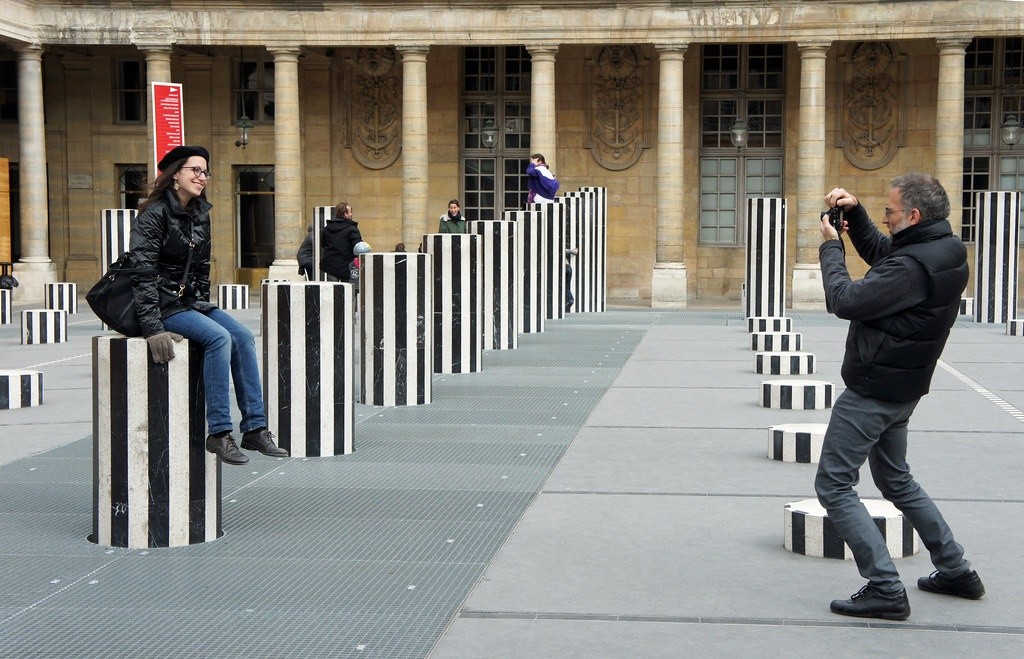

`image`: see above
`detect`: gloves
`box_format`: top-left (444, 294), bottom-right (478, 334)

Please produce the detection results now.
top-left (147, 331), bottom-right (184, 363)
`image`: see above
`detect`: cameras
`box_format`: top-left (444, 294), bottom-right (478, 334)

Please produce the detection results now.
top-left (821, 205), bottom-right (844, 232)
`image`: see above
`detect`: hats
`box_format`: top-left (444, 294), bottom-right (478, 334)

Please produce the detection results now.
top-left (156, 145), bottom-right (209, 172)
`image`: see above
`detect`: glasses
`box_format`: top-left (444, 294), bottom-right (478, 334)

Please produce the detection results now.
top-left (885, 208), bottom-right (913, 217)
top-left (181, 166), bottom-right (212, 180)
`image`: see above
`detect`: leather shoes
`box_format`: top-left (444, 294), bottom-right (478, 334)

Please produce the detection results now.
top-left (830, 585), bottom-right (910, 619)
top-left (240, 427), bottom-right (288, 456)
top-left (206, 434), bottom-right (248, 465)
top-left (917, 569), bottom-right (985, 599)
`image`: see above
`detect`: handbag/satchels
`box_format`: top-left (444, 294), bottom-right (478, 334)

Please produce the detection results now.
top-left (85, 251), bottom-right (184, 337)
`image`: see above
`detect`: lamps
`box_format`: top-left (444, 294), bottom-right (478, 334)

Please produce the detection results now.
top-left (729, 113), bottom-right (752, 159)
top-left (1000, 115), bottom-right (1024, 152)
top-left (480, 117), bottom-right (503, 152)
top-left (235, 114), bottom-right (254, 148)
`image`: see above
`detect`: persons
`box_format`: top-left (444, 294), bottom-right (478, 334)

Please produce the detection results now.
top-left (321, 202), bottom-right (372, 311)
top-left (296, 225), bottom-right (313, 280)
top-left (565, 248), bottom-right (578, 309)
top-left (527, 154), bottom-right (559, 204)
top-left (129, 146), bottom-right (288, 466)
top-left (438, 200), bottom-right (466, 234)
top-left (818, 173), bottom-right (987, 621)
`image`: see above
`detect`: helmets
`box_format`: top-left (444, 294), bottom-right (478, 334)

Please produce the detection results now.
top-left (353, 242), bottom-right (371, 254)
